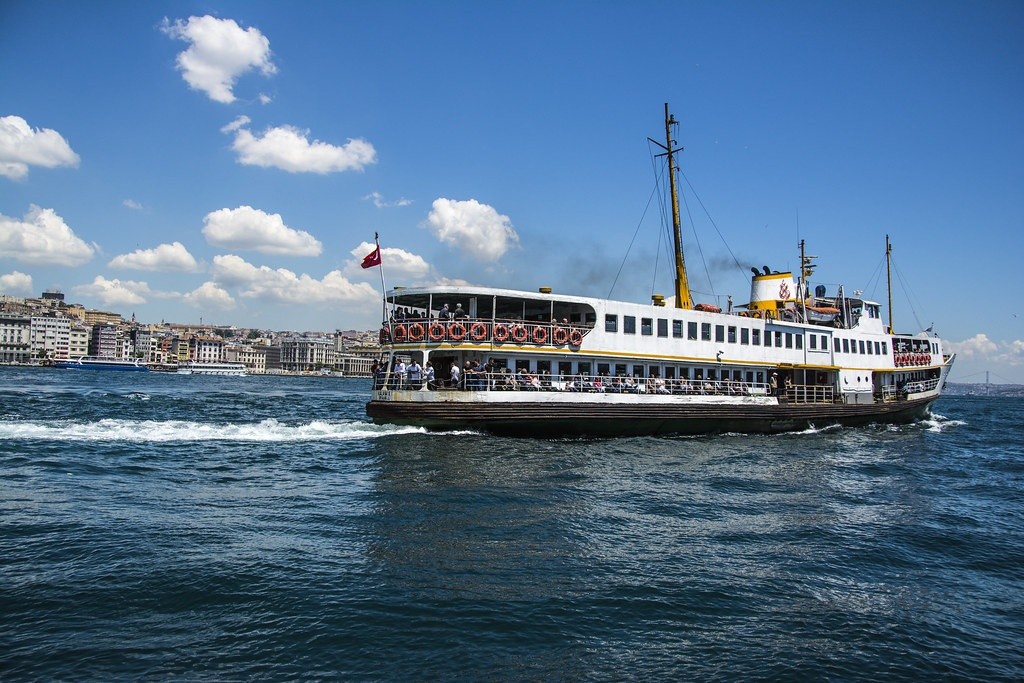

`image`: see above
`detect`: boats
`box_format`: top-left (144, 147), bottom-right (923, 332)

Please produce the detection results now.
top-left (362, 104), bottom-right (956, 423)
top-left (805, 309), bottom-right (840, 324)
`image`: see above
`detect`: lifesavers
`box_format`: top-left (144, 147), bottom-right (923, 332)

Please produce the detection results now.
top-left (570, 330), bottom-right (583, 346)
top-left (920, 355), bottom-right (925, 364)
top-left (911, 355), bottom-right (916, 363)
top-left (900, 355), bottom-right (906, 364)
top-left (554, 328), bottom-right (568, 345)
top-left (429, 324), bottom-right (445, 340)
top-left (915, 354), bottom-right (920, 364)
top-left (409, 324), bottom-right (424, 340)
top-left (905, 355), bottom-right (911, 362)
top-left (924, 355), bottom-right (929, 363)
top-left (512, 325), bottom-right (527, 342)
top-left (471, 324), bottom-right (486, 340)
top-left (895, 356), bottom-right (901, 364)
top-left (494, 325), bottom-right (509, 341)
top-left (533, 327), bottom-right (547, 345)
top-left (393, 326), bottom-right (406, 342)
top-left (928, 354), bottom-right (931, 362)
top-left (449, 324), bottom-right (466, 340)
top-left (752, 311), bottom-right (760, 318)
top-left (435, 363), bottom-right (442, 370)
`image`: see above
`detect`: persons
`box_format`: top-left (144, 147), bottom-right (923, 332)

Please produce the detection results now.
top-left (770, 372), bottom-right (779, 396)
top-left (503, 314), bottom-right (521, 323)
top-left (438, 304), bottom-right (450, 340)
top-left (450, 362), bottom-right (459, 388)
top-left (649, 373), bottom-right (751, 396)
top-left (784, 373), bottom-right (792, 398)
top-left (566, 372), bottom-right (641, 394)
top-left (390, 307), bottom-right (434, 328)
top-left (900, 370), bottom-right (935, 388)
top-left (371, 356), bottom-right (439, 390)
top-left (454, 303), bottom-right (468, 339)
top-left (916, 347), bottom-right (921, 353)
top-left (854, 309), bottom-right (860, 314)
top-left (463, 357), bottom-right (558, 392)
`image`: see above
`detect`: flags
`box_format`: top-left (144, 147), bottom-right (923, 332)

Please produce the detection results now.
top-left (361, 245), bottom-right (381, 269)
top-left (925, 326), bottom-right (932, 332)
